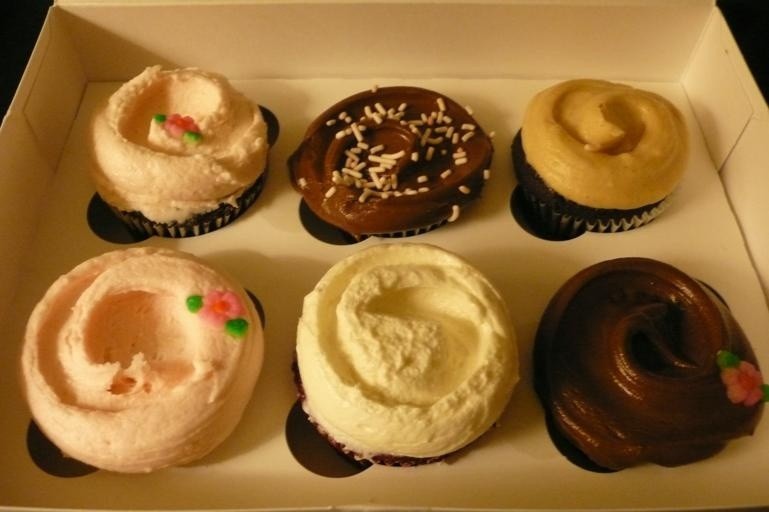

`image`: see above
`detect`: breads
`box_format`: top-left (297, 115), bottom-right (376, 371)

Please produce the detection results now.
top-left (21, 65), bottom-right (769, 475)
